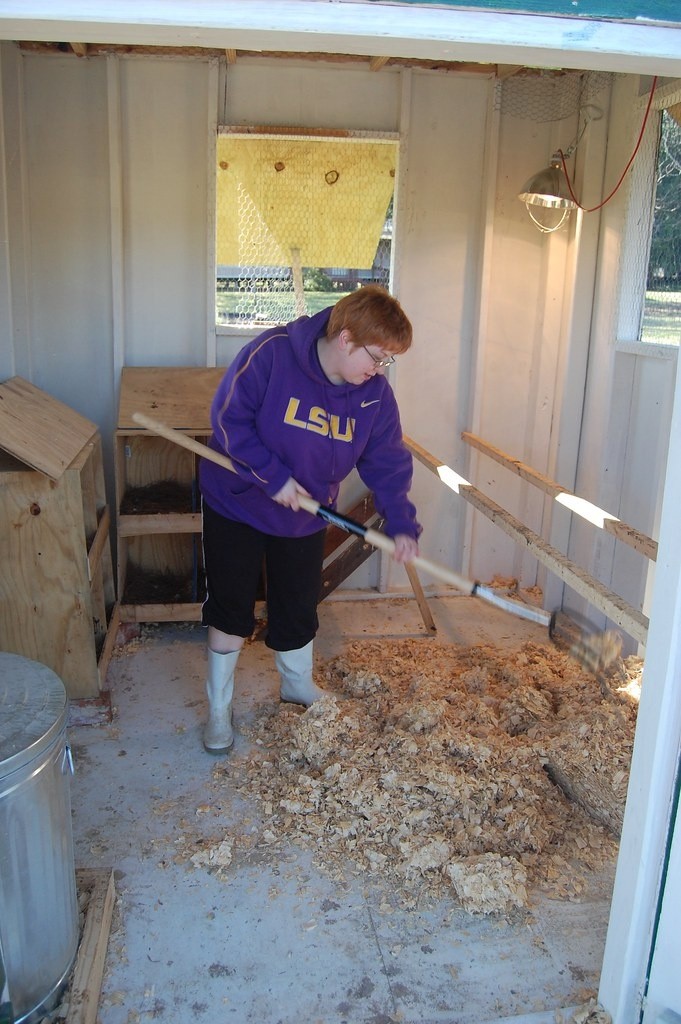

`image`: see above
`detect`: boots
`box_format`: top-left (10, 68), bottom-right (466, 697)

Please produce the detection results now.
top-left (203, 647), bottom-right (242, 753)
top-left (274, 638), bottom-right (348, 707)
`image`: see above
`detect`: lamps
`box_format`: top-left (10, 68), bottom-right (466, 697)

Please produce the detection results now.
top-left (518, 147), bottom-right (579, 233)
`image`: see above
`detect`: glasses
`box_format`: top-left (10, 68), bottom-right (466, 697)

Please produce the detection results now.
top-left (362, 344), bottom-right (396, 368)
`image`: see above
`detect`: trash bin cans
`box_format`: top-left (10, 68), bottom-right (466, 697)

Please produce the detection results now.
top-left (1, 649), bottom-right (87, 1024)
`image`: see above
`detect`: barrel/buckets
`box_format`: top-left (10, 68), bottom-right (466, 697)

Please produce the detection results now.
top-left (0, 649), bottom-right (83, 1024)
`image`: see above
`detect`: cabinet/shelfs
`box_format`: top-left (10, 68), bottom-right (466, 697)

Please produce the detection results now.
top-left (0, 429), bottom-right (268, 700)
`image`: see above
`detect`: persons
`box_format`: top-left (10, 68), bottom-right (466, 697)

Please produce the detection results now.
top-left (199, 283), bottom-right (423, 757)
